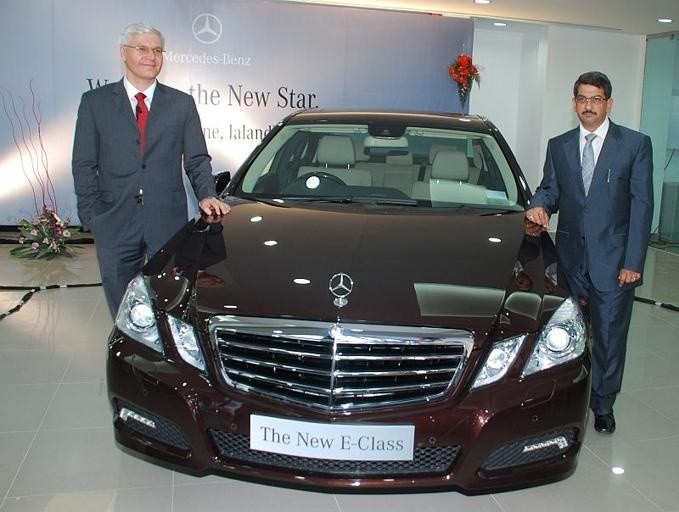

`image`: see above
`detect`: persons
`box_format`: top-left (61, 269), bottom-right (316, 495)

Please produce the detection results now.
top-left (142, 215), bottom-right (228, 288)
top-left (526, 71), bottom-right (654, 435)
top-left (72, 22), bottom-right (231, 324)
top-left (539, 232), bottom-right (568, 295)
top-left (511, 236), bottom-right (540, 291)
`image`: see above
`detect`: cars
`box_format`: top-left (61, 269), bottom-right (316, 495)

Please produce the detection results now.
top-left (104, 106), bottom-right (597, 495)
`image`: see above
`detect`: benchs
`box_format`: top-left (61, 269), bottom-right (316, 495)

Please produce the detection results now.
top-left (349, 140), bottom-right (481, 197)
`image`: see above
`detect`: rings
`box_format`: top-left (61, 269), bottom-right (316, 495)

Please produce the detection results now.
top-left (631, 277), bottom-right (637, 280)
top-left (529, 215), bottom-right (534, 219)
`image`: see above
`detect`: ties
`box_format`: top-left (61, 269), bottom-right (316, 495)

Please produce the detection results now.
top-left (135, 92), bottom-right (148, 158)
top-left (582, 134), bottom-right (597, 198)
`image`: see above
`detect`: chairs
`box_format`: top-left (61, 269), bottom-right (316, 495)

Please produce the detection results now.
top-left (409, 150), bottom-right (491, 207)
top-left (298, 134), bottom-right (373, 188)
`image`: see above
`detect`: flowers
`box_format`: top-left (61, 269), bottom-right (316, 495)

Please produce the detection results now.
top-left (10, 202), bottom-right (85, 265)
top-left (449, 50), bottom-right (483, 112)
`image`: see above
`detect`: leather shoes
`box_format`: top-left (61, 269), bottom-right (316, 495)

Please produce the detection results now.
top-left (596, 412), bottom-right (615, 434)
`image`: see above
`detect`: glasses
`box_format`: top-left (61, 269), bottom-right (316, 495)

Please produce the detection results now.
top-left (124, 45), bottom-right (166, 55)
top-left (577, 95), bottom-right (606, 104)
